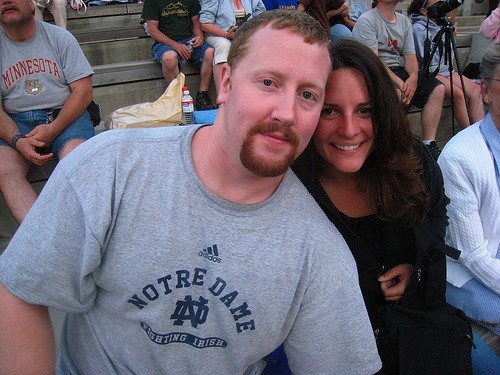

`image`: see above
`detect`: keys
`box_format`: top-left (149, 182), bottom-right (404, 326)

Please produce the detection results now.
top-left (46, 112), bottom-right (53, 124)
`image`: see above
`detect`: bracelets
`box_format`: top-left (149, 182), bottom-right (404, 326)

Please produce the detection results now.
top-left (12, 134), bottom-right (26, 148)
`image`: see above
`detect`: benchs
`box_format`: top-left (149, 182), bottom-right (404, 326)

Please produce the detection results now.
top-left (0, 0), bottom-right (500, 359)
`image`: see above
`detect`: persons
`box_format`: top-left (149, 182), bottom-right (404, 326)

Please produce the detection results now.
top-left (436, 42), bottom-right (500, 337)
top-left (0, 10), bottom-right (382, 375)
top-left (0, 0), bottom-right (95, 225)
top-left (141, 0), bottom-right (500, 162)
top-left (290, 39), bottom-right (473, 375)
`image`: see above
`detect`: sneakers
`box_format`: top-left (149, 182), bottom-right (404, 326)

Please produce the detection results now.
top-left (425, 140), bottom-right (441, 159)
top-left (196, 89), bottom-right (215, 109)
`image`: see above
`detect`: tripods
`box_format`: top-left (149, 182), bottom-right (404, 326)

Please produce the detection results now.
top-left (407, 17), bottom-right (473, 136)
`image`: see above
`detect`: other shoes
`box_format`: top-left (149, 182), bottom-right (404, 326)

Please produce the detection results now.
top-left (88, 0), bottom-right (109, 7)
top-left (101, 0), bottom-right (129, 3)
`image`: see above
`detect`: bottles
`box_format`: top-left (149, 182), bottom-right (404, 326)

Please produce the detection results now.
top-left (181, 86), bottom-right (188, 124)
top-left (182, 42), bottom-right (193, 65)
top-left (182, 90), bottom-right (195, 124)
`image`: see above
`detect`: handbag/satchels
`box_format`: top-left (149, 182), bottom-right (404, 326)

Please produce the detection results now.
top-left (66, 80), bottom-right (102, 128)
